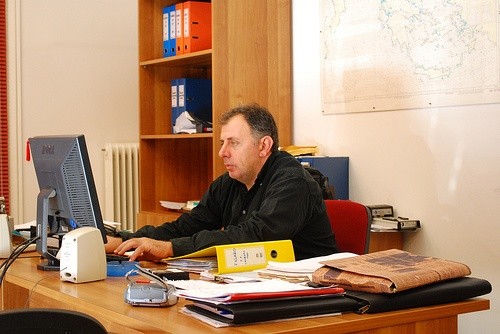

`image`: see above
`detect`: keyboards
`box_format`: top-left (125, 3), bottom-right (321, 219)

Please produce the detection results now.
top-left (105, 253), bottom-right (139, 263)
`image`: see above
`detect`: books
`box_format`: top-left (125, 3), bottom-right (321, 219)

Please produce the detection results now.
top-left (182, 248), bottom-right (491, 327)
top-left (366, 205), bottom-right (421, 230)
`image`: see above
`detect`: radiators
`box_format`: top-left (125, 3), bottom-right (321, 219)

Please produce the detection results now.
top-left (101, 143), bottom-right (140, 233)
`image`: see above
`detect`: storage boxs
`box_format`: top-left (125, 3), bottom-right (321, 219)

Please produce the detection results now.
top-left (296, 155), bottom-right (350, 199)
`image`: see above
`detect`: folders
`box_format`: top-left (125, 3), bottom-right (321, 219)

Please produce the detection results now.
top-left (164, 239), bottom-right (295, 272)
top-left (171, 78), bottom-right (212, 133)
top-left (162, 1), bottom-right (212, 57)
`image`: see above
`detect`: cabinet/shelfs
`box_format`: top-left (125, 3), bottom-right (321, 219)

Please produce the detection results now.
top-left (135, 0), bottom-right (292, 230)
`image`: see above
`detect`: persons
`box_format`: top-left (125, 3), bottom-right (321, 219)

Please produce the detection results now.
top-left (105, 104), bottom-right (339, 261)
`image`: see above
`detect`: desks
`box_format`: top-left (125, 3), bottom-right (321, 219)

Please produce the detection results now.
top-left (0, 233), bottom-right (491, 334)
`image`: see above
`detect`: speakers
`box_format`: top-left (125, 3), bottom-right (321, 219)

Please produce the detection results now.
top-left (0, 214), bottom-right (12, 258)
top-left (60, 226), bottom-right (107, 283)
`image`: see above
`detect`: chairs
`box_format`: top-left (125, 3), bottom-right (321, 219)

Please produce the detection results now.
top-left (325, 199), bottom-right (372, 255)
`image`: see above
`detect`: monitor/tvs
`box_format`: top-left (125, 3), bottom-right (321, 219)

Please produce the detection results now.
top-left (27, 133), bottom-right (108, 272)
